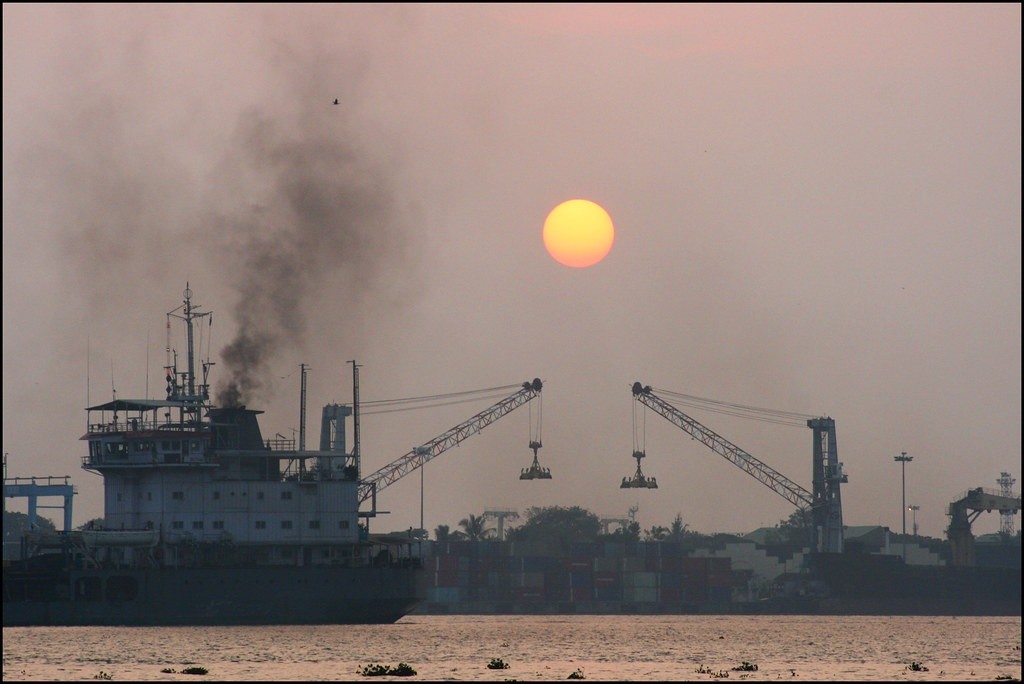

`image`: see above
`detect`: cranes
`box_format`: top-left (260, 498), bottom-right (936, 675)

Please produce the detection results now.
top-left (620, 381), bottom-right (849, 525)
top-left (317, 378), bottom-right (551, 507)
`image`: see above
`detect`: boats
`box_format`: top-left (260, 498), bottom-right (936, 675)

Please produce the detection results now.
top-left (1, 280), bottom-right (427, 626)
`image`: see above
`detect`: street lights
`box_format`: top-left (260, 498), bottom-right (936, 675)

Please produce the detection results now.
top-left (894, 452), bottom-right (913, 564)
top-left (908, 505), bottom-right (919, 535)
